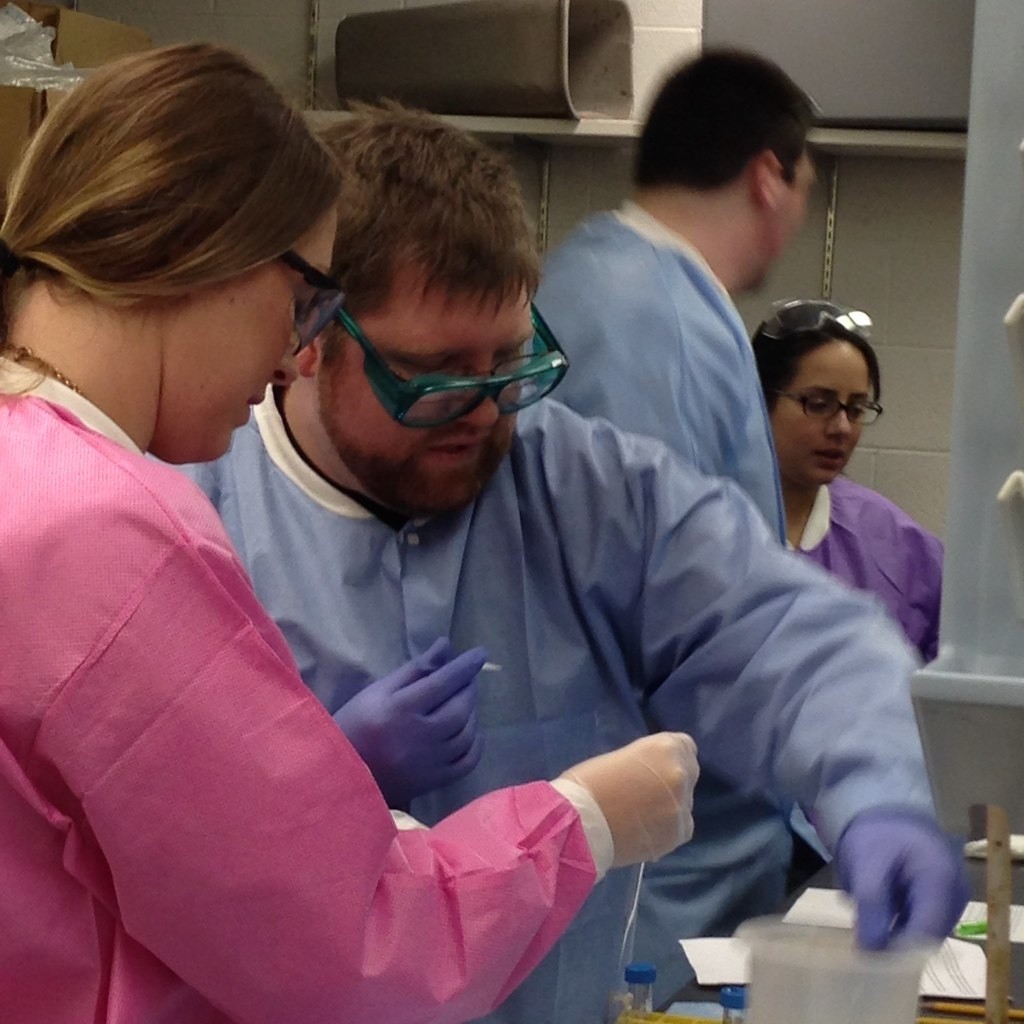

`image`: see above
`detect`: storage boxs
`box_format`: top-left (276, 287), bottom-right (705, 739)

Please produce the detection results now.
top-left (0, 0), bottom-right (153, 216)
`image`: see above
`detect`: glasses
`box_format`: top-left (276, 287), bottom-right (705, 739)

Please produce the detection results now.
top-left (769, 388), bottom-right (883, 425)
top-left (333, 301), bottom-right (569, 427)
top-left (278, 248), bottom-right (346, 355)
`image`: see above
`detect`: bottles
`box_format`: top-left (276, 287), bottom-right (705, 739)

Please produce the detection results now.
top-left (719, 987), bottom-right (749, 1024)
top-left (624, 962), bottom-right (656, 1012)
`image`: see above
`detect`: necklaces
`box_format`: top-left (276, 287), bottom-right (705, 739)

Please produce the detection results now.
top-left (6, 343), bottom-right (83, 394)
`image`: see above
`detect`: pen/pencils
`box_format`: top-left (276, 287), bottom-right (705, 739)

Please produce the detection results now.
top-left (957, 920), bottom-right (989, 934)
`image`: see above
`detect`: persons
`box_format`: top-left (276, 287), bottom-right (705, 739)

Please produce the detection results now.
top-left (514, 46), bottom-right (823, 1009)
top-left (177, 102), bottom-right (968, 1024)
top-left (754, 300), bottom-right (944, 663)
top-left (0, 41), bottom-right (699, 1024)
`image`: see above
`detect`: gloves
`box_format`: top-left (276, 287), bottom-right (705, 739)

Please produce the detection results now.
top-left (330, 636), bottom-right (487, 809)
top-left (836, 804), bottom-right (972, 966)
